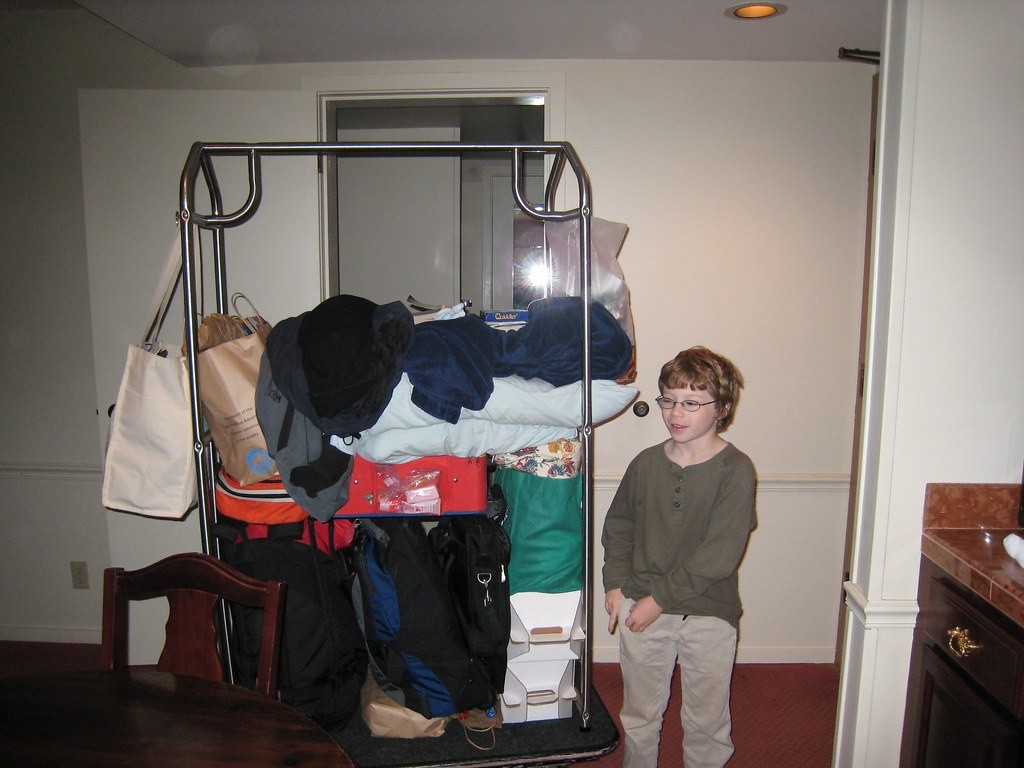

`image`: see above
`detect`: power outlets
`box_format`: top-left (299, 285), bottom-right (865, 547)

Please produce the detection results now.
top-left (70, 561), bottom-right (90, 590)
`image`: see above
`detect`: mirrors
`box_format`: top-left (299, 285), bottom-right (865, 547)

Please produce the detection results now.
top-left (314, 86), bottom-right (551, 319)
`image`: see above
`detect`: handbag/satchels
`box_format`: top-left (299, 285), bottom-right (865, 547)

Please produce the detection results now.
top-left (100, 212), bottom-right (199, 519)
top-left (430, 514), bottom-right (511, 693)
top-left (456, 698), bottom-right (503, 750)
top-left (266, 293), bottom-right (415, 445)
top-left (513, 206), bottom-right (636, 385)
top-left (360, 663), bottom-right (457, 739)
top-left (214, 463), bottom-right (311, 525)
top-left (183, 292), bottom-right (279, 487)
top-left (492, 453), bottom-right (585, 595)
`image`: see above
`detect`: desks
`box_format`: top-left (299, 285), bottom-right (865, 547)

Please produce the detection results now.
top-left (0, 671), bottom-right (354, 768)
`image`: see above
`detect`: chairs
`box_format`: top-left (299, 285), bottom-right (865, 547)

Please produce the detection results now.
top-left (100, 552), bottom-right (287, 699)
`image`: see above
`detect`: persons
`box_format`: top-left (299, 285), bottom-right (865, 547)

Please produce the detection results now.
top-left (602, 346), bottom-right (758, 768)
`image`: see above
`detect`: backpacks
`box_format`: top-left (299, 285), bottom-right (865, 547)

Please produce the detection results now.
top-left (255, 348), bottom-right (354, 523)
top-left (211, 526), bottom-right (368, 730)
top-left (340, 517), bottom-right (496, 720)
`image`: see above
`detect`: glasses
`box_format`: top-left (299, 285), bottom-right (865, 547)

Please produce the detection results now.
top-left (655, 394), bottom-right (719, 412)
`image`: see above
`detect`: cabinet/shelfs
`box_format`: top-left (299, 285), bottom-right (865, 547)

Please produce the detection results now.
top-left (898, 554), bottom-right (1024, 768)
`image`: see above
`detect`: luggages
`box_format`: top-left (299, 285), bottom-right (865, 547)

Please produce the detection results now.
top-left (311, 449), bottom-right (508, 521)
